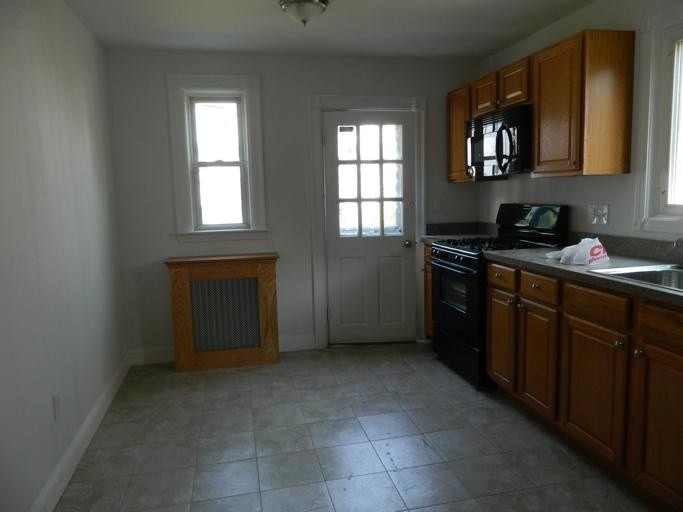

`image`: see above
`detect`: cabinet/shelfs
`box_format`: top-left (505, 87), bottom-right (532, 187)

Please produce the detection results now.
top-left (421, 246), bottom-right (433, 339)
top-left (447, 82), bottom-right (475, 184)
top-left (470, 56), bottom-right (533, 119)
top-left (557, 279), bottom-right (683, 510)
top-left (531, 28), bottom-right (636, 178)
top-left (485, 261), bottom-right (560, 432)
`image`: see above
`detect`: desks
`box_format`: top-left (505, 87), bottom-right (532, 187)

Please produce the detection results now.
top-left (164, 253), bottom-right (279, 373)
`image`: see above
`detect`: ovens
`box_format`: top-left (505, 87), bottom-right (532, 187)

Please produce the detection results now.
top-left (431, 246), bottom-right (486, 381)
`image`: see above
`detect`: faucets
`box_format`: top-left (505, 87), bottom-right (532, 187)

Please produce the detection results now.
top-left (674, 237), bottom-right (683, 248)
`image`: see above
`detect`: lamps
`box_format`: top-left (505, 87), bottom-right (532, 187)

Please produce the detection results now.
top-left (278, 0), bottom-right (330, 27)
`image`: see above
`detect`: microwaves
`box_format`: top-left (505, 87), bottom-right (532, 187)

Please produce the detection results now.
top-left (465, 105), bottom-right (530, 180)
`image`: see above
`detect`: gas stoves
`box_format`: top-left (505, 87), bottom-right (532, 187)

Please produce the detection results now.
top-left (431, 237), bottom-right (561, 255)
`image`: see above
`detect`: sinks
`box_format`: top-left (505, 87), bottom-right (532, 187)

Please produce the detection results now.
top-left (586, 264), bottom-right (683, 294)
